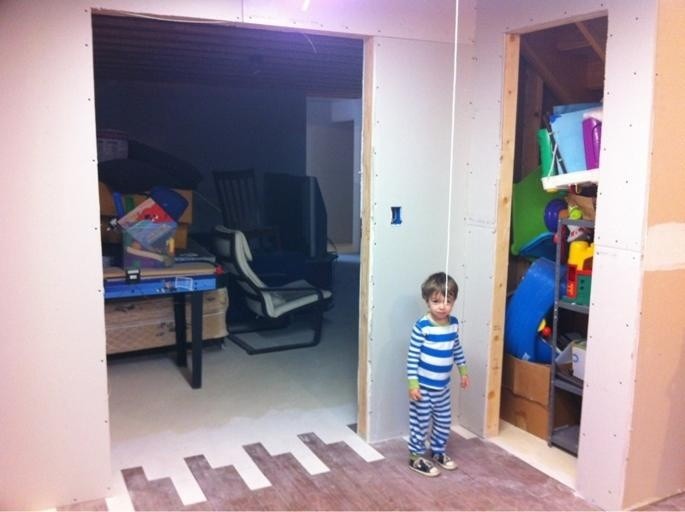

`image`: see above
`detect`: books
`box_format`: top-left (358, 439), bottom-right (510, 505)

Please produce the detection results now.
top-left (126, 243), bottom-right (175, 266)
top-left (118, 196), bottom-right (177, 247)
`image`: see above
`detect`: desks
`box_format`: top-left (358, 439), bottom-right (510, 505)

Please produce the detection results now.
top-left (102, 260), bottom-right (228, 389)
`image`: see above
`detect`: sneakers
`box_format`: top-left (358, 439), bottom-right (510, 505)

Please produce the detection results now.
top-left (433, 453), bottom-right (458, 471)
top-left (409, 455), bottom-right (439, 477)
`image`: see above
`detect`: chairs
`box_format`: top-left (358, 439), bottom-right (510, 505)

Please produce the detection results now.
top-left (203, 169), bottom-right (336, 355)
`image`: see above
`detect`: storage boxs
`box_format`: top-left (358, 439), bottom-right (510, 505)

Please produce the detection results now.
top-left (510, 163), bottom-right (562, 263)
top-left (120, 219), bottom-right (178, 270)
top-left (499, 349), bottom-right (581, 440)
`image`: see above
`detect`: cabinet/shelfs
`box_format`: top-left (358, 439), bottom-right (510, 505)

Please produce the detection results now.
top-left (547, 217), bottom-right (596, 456)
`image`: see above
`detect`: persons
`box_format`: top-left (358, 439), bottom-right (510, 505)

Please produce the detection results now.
top-left (405, 272), bottom-right (471, 477)
